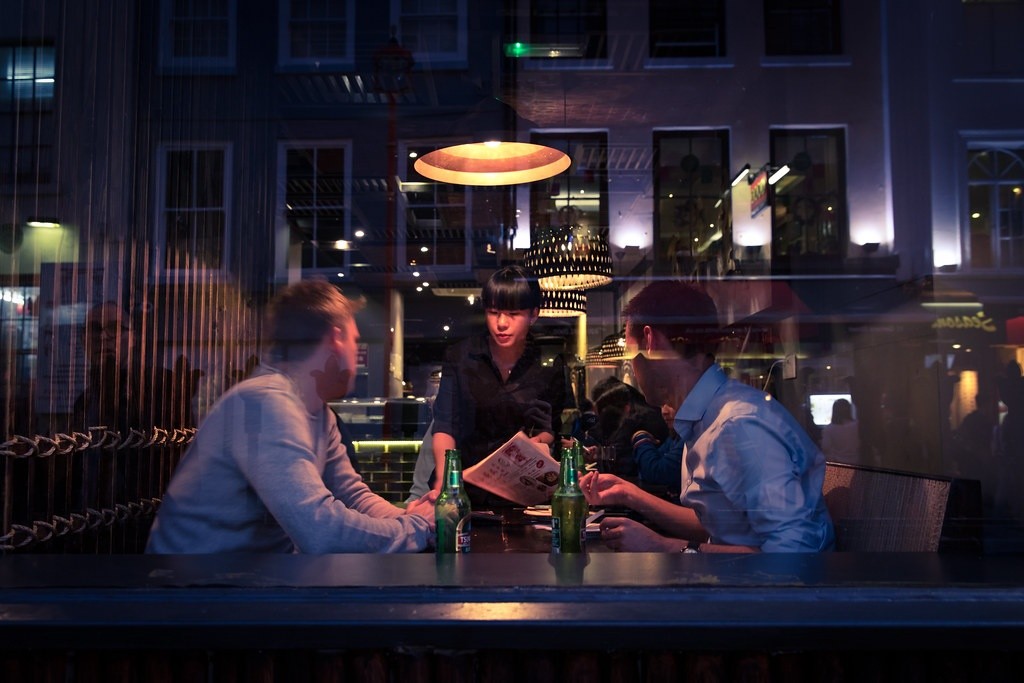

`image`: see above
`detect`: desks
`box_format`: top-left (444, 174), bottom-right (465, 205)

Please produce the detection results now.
top-left (471, 505), bottom-right (616, 552)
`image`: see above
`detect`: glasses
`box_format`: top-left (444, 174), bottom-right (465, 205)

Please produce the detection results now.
top-left (81, 319), bottom-right (130, 338)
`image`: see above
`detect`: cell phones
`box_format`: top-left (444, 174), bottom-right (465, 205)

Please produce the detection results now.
top-left (471, 514), bottom-right (503, 528)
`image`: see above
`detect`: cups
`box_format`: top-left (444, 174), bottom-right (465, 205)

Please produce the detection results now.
top-left (598, 446), bottom-right (616, 472)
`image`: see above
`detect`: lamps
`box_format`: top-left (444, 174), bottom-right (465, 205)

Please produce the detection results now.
top-left (522, 90), bottom-right (615, 292)
top-left (413, 98), bottom-right (572, 186)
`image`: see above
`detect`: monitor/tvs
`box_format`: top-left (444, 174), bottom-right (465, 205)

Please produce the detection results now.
top-left (808, 392), bottom-right (854, 427)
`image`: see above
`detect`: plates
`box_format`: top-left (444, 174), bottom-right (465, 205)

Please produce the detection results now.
top-left (524, 506), bottom-right (551, 520)
top-left (532, 522), bottom-right (603, 538)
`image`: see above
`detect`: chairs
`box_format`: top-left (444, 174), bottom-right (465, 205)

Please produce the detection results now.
top-left (822, 460), bottom-right (982, 553)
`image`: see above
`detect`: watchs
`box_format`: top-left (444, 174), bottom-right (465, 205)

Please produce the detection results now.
top-left (680, 540), bottom-right (702, 553)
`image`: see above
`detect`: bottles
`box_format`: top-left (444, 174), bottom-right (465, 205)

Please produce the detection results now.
top-left (435, 449), bottom-right (472, 553)
top-left (572, 442), bottom-right (589, 528)
top-left (552, 447), bottom-right (586, 553)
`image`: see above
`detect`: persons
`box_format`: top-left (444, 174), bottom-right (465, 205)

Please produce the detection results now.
top-left (48, 300), bottom-right (191, 550)
top-left (561, 376), bottom-right (684, 504)
top-left (577, 280), bottom-right (836, 553)
top-left (146, 279), bottom-right (459, 558)
top-left (824, 363), bottom-right (1024, 491)
top-left (427, 266), bottom-right (567, 502)
